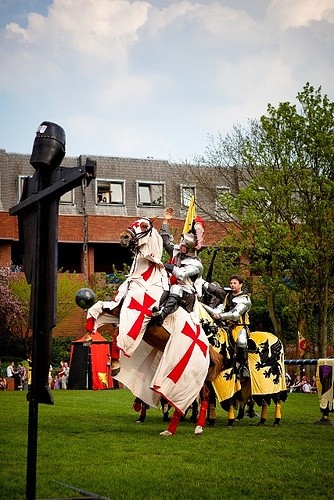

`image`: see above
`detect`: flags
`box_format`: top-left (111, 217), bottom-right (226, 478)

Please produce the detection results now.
top-left (298, 331), bottom-right (311, 359)
top-left (180, 194), bottom-right (198, 243)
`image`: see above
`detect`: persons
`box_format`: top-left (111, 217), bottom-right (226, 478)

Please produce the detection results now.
top-left (48, 361), bottom-right (70, 390)
top-left (313, 344), bottom-right (334, 426)
top-left (7, 361), bottom-right (27, 390)
top-left (202, 275), bottom-right (252, 378)
top-left (151, 207), bottom-right (204, 326)
top-left (100, 197), bottom-right (106, 204)
top-left (285, 371), bottom-right (318, 394)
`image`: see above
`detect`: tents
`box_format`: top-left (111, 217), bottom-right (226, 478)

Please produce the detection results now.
top-left (66, 329), bottom-right (115, 390)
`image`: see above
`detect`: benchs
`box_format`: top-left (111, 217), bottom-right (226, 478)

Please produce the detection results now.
top-left (6, 378), bottom-right (18, 390)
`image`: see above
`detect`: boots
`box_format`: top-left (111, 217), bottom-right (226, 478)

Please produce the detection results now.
top-left (152, 293), bottom-right (181, 327)
top-left (238, 347), bottom-right (250, 377)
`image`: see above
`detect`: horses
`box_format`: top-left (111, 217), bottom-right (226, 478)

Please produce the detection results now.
top-left (135, 331), bottom-right (287, 427)
top-left (86, 216), bottom-right (226, 437)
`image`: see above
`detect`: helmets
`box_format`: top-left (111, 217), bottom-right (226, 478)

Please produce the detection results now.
top-left (181, 232), bottom-right (198, 249)
